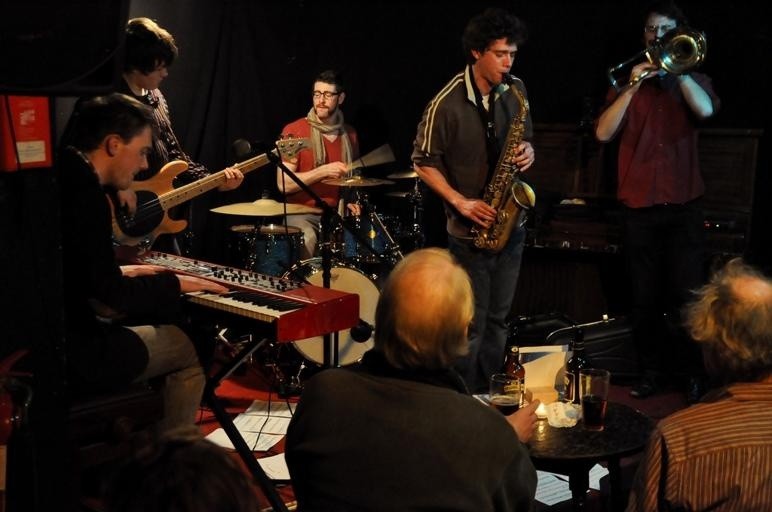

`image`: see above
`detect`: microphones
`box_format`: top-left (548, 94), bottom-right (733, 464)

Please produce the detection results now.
top-left (231, 138), bottom-right (269, 157)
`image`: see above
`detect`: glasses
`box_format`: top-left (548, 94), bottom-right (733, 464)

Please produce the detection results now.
top-left (313, 90), bottom-right (341, 99)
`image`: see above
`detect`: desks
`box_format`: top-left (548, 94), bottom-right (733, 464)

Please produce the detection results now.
top-left (496, 394), bottom-right (659, 510)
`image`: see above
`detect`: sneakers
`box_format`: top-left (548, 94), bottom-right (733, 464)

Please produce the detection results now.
top-left (685, 378), bottom-right (701, 405)
top-left (630, 378), bottom-right (665, 398)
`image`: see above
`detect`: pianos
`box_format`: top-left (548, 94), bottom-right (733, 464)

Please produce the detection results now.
top-left (123, 252), bottom-right (363, 346)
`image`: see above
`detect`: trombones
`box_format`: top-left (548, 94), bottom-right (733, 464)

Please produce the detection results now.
top-left (607, 26), bottom-right (708, 92)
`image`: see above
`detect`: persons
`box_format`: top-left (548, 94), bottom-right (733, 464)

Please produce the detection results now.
top-left (58, 16), bottom-right (245, 261)
top-left (288, 249), bottom-right (543, 511)
top-left (113, 439), bottom-right (258, 510)
top-left (595, 9), bottom-right (727, 397)
top-left (10, 91), bottom-right (230, 440)
top-left (623, 261), bottom-right (770, 512)
top-left (276, 71), bottom-right (370, 265)
top-left (409, 16), bottom-right (536, 394)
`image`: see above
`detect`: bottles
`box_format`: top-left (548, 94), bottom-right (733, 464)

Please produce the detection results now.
top-left (563, 338), bottom-right (591, 404)
top-left (500, 344), bottom-right (526, 407)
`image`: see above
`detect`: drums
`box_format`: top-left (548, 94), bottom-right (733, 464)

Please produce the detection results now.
top-left (343, 212), bottom-right (406, 266)
top-left (230, 226), bottom-right (304, 275)
top-left (272, 258), bottom-right (384, 369)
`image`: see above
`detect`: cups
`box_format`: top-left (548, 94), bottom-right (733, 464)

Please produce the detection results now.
top-left (578, 367), bottom-right (611, 432)
top-left (488, 372), bottom-right (520, 416)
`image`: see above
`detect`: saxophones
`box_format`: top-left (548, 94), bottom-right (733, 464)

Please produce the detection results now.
top-left (470, 73), bottom-right (539, 251)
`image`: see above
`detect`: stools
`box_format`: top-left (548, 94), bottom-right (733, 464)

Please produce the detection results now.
top-left (8, 370), bottom-right (168, 508)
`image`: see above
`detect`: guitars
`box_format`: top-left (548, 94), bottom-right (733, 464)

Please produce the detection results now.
top-left (108, 133), bottom-right (311, 251)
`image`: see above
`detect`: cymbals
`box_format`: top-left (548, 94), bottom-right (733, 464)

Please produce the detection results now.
top-left (210, 197), bottom-right (324, 217)
top-left (394, 170), bottom-right (420, 180)
top-left (320, 174), bottom-right (391, 187)
